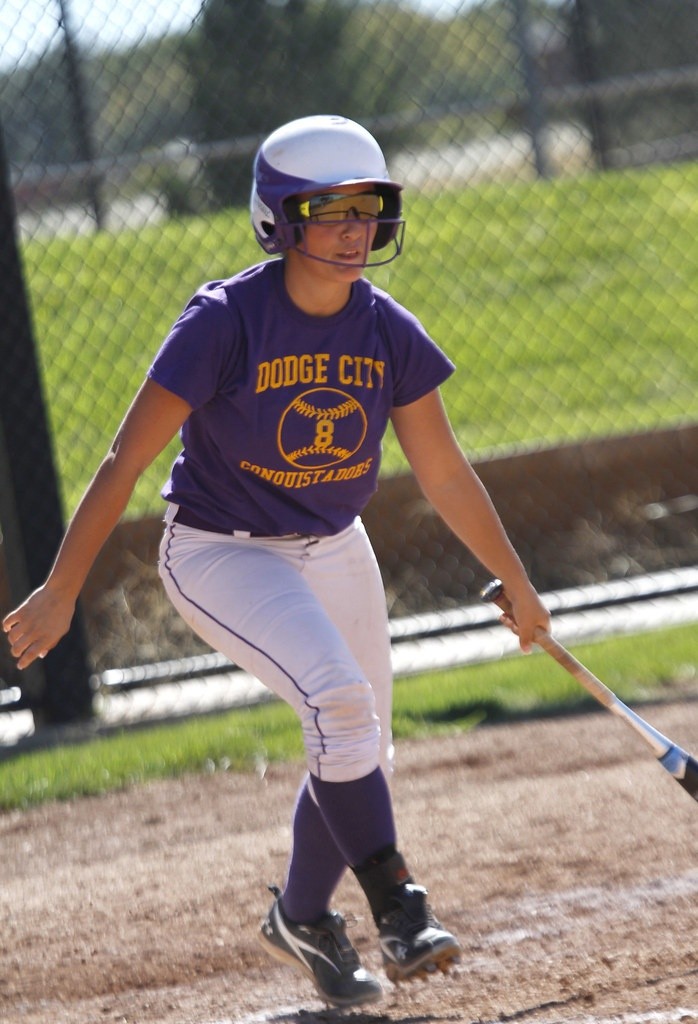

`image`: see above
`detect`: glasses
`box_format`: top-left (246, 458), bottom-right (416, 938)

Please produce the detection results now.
top-left (286, 193), bottom-right (385, 225)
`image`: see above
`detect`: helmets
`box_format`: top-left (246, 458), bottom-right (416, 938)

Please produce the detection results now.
top-left (249, 115), bottom-right (404, 254)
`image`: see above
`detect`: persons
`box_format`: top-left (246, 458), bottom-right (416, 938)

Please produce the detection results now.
top-left (2, 117), bottom-right (557, 1004)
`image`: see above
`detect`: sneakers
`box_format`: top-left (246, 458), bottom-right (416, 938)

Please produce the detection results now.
top-left (253, 888), bottom-right (385, 1005)
top-left (376, 884), bottom-right (461, 985)
